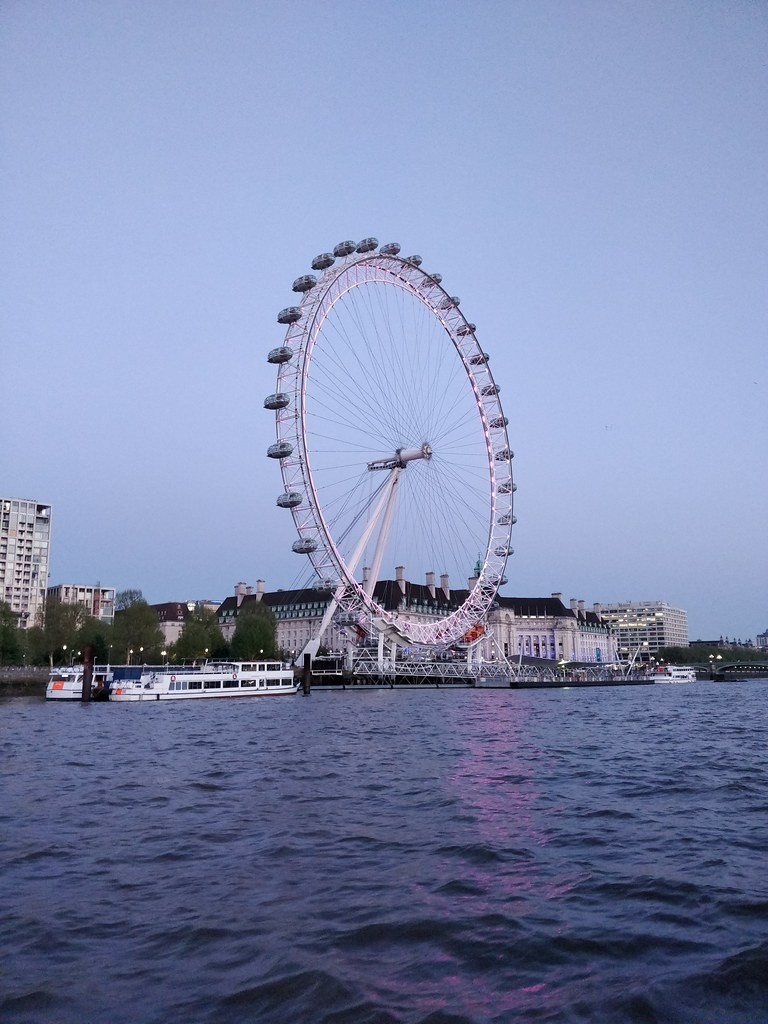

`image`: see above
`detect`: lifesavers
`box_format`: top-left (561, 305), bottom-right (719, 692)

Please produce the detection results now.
top-left (233, 673), bottom-right (237, 679)
top-left (170, 675), bottom-right (176, 682)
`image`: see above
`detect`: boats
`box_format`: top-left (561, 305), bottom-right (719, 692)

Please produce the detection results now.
top-left (109, 659), bottom-right (301, 702)
top-left (45, 663), bottom-right (204, 701)
top-left (654, 665), bottom-right (697, 684)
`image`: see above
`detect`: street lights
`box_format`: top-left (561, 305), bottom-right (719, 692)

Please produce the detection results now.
top-left (108, 644), bottom-right (112, 658)
top-left (161, 651), bottom-right (167, 665)
top-left (130, 649), bottom-right (133, 665)
top-left (70, 649), bottom-right (74, 666)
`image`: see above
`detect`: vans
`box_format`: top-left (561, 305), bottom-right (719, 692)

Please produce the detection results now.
top-left (613, 660), bottom-right (632, 670)
